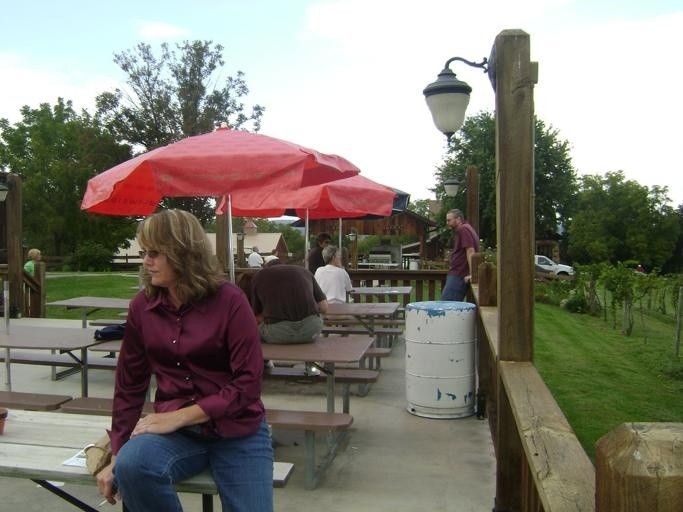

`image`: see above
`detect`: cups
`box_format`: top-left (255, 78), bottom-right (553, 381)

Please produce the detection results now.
top-left (359, 279), bottom-right (366, 289)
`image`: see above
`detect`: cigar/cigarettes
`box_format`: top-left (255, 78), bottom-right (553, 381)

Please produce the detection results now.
top-left (98, 496), bottom-right (114, 508)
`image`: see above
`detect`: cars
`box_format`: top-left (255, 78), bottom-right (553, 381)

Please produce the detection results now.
top-left (534, 253), bottom-right (574, 277)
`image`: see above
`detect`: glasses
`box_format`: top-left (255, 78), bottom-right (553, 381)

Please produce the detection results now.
top-left (138, 250), bottom-right (167, 259)
top-left (320, 239), bottom-right (330, 244)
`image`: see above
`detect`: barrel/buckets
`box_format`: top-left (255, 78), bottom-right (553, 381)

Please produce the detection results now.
top-left (405, 301), bottom-right (478, 419)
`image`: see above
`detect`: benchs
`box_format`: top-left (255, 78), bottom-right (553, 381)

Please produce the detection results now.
top-left (258, 287), bottom-right (414, 487)
top-left (0, 288), bottom-right (131, 512)
top-left (175, 466), bottom-right (221, 512)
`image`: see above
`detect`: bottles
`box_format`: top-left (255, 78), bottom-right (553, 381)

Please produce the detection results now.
top-left (401, 257), bottom-right (415, 270)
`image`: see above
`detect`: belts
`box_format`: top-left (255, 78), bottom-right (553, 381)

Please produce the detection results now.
top-left (264, 311), bottom-right (320, 323)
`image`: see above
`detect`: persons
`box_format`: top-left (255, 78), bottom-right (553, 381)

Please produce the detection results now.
top-left (24, 249), bottom-right (41, 309)
top-left (304, 233), bottom-right (331, 275)
top-left (247, 246), bottom-right (264, 268)
top-left (439, 208), bottom-right (479, 301)
top-left (313, 245), bottom-right (352, 337)
top-left (637, 265), bottom-right (644, 273)
top-left (251, 255), bottom-right (328, 376)
top-left (96, 208), bottom-right (274, 511)
top-left (264, 249), bottom-right (279, 265)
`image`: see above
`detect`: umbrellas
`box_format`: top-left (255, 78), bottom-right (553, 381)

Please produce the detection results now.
top-left (81, 126), bottom-right (361, 287)
top-left (215, 173), bottom-right (396, 270)
top-left (284, 187), bottom-right (411, 262)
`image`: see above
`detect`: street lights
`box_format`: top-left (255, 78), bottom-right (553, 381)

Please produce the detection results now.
top-left (444, 162), bottom-right (481, 250)
top-left (348, 227), bottom-right (358, 270)
top-left (0, 172), bottom-right (24, 317)
top-left (235, 231), bottom-right (246, 262)
top-left (422, 27), bottom-right (540, 364)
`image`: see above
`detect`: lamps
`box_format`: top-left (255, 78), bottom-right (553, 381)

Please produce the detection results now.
top-left (0, 181), bottom-right (9, 202)
top-left (419, 44), bottom-right (496, 150)
top-left (442, 172), bottom-right (468, 199)
top-left (348, 229), bottom-right (356, 241)
